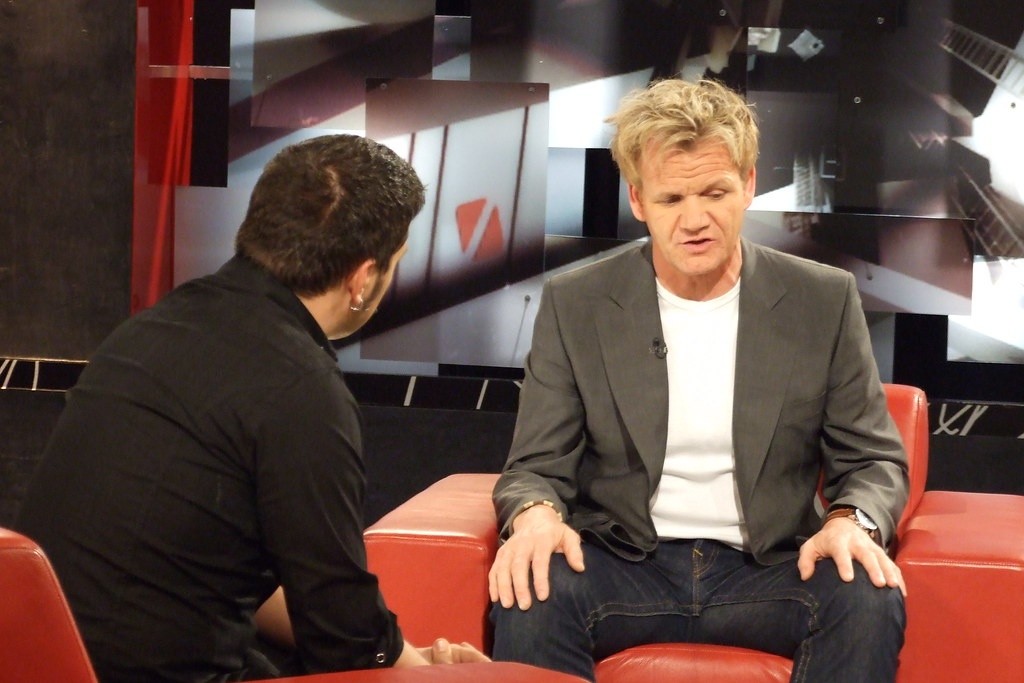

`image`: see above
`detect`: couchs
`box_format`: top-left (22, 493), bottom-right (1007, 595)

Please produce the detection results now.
top-left (1, 529), bottom-right (592, 683)
top-left (361, 382), bottom-right (1024, 682)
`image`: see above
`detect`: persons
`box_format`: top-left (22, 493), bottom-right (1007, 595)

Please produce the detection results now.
top-left (16, 133), bottom-right (492, 683)
top-left (487, 77), bottom-right (909, 683)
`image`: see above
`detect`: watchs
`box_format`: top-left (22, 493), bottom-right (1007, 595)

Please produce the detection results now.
top-left (823, 506), bottom-right (882, 537)
